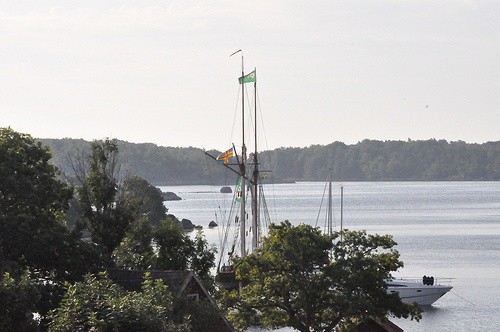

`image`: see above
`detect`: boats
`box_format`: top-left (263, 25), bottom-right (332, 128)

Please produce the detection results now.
top-left (298, 168), bottom-right (454, 308)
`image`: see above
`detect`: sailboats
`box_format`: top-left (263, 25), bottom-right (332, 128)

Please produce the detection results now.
top-left (199, 49), bottom-right (274, 291)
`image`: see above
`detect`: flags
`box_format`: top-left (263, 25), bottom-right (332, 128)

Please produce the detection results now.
top-left (216, 147), bottom-right (234, 161)
top-left (238, 70), bottom-right (256, 84)
